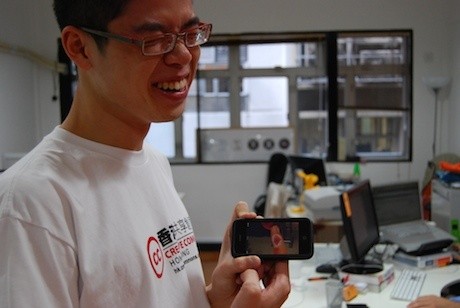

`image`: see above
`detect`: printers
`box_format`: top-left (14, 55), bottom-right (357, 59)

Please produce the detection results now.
top-left (304, 184), bottom-right (352, 221)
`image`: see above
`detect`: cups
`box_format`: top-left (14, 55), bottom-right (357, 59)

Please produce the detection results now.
top-left (325, 281), bottom-right (344, 308)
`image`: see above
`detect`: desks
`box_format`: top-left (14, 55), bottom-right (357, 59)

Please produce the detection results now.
top-left (282, 243), bottom-right (460, 308)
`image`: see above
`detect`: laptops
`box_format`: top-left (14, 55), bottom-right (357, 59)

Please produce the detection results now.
top-left (372, 181), bottom-right (456, 253)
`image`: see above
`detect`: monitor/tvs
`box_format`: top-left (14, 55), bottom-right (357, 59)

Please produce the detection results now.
top-left (288, 156), bottom-right (328, 193)
top-left (336, 180), bottom-right (384, 274)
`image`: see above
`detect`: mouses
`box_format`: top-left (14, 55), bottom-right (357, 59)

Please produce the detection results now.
top-left (316, 264), bottom-right (337, 273)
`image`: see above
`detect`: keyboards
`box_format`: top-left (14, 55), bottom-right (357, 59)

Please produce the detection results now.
top-left (391, 269), bottom-right (426, 301)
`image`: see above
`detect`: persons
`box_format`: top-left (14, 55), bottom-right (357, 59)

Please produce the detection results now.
top-left (270, 226), bottom-right (286, 253)
top-left (407, 294), bottom-right (460, 308)
top-left (0, 0), bottom-right (291, 308)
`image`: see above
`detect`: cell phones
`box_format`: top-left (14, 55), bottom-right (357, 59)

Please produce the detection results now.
top-left (231, 218), bottom-right (314, 260)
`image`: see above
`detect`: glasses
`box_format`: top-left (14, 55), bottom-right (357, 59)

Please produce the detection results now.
top-left (79, 20), bottom-right (214, 58)
